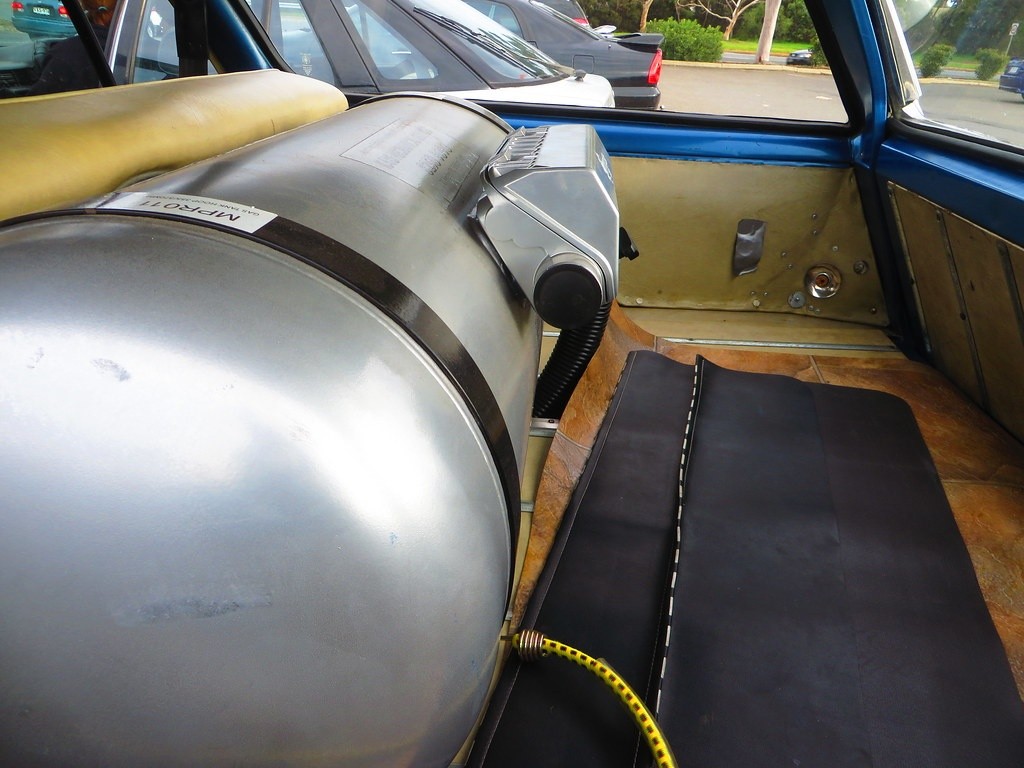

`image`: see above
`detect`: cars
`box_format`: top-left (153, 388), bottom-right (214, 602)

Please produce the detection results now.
top-left (999, 56), bottom-right (1024, 102)
top-left (9, 0), bottom-right (80, 43)
top-left (786, 50), bottom-right (818, 67)
top-left (100, 0), bottom-right (614, 117)
top-left (249, 0), bottom-right (666, 117)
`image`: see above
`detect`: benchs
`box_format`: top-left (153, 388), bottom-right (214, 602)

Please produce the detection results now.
top-left (0, 65), bottom-right (355, 223)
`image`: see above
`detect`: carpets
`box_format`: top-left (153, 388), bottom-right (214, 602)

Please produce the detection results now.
top-left (464, 348), bottom-right (1024, 768)
